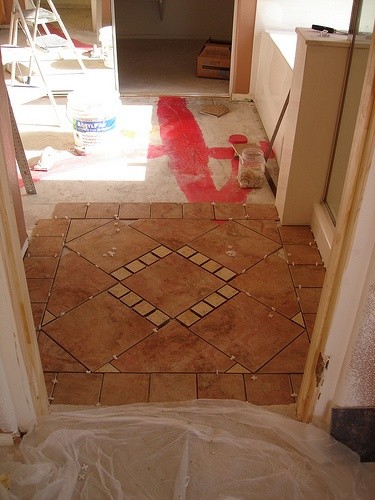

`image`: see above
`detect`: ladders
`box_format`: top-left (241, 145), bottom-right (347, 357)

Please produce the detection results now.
top-left (7, 0), bottom-right (90, 123)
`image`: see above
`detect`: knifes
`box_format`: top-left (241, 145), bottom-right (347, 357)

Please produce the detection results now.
top-left (312, 24), bottom-right (349, 35)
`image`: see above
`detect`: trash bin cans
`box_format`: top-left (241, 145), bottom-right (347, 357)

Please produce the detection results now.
top-left (330, 407), bottom-right (375, 463)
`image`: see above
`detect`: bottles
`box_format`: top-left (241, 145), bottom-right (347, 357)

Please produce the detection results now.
top-left (237, 148), bottom-right (265, 189)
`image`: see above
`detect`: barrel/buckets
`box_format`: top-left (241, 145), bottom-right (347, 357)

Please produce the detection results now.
top-left (66, 88), bottom-right (123, 156)
top-left (98, 25), bottom-right (115, 68)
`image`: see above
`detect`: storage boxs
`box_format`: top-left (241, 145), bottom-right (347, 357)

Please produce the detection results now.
top-left (194, 38), bottom-right (231, 80)
